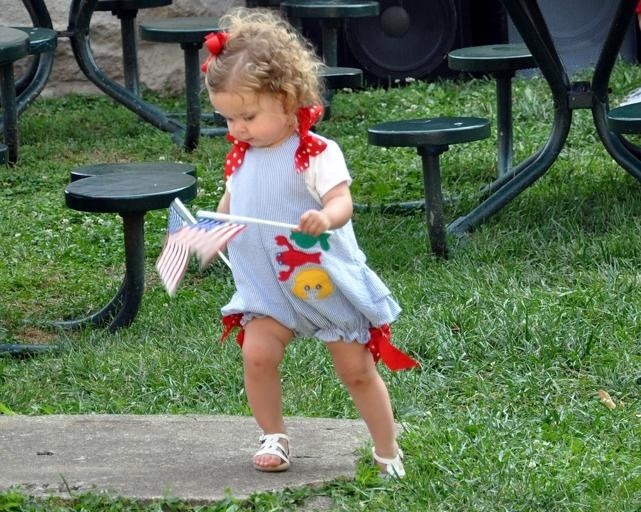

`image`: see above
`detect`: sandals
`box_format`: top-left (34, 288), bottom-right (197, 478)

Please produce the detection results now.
top-left (252, 431), bottom-right (291, 474)
top-left (370, 444), bottom-right (407, 485)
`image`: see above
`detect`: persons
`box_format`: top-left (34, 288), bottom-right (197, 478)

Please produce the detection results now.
top-left (203, 6), bottom-right (420, 479)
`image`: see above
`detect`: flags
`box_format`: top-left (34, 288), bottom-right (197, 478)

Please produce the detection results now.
top-left (155, 201), bottom-right (194, 298)
top-left (170, 218), bottom-right (248, 267)
